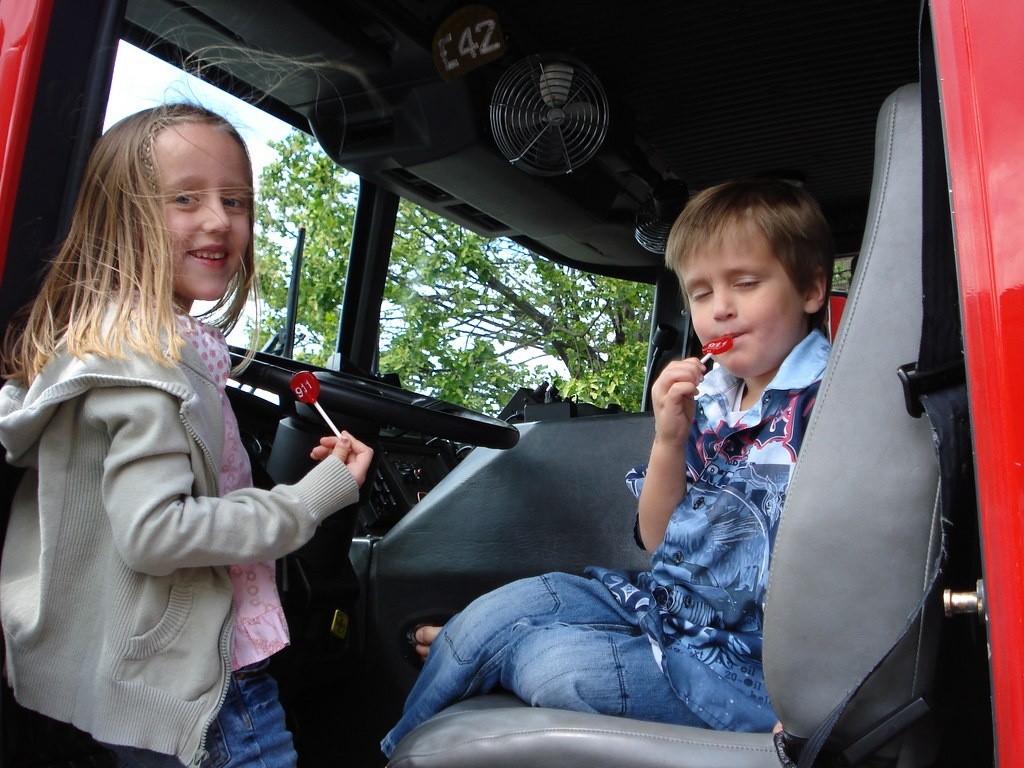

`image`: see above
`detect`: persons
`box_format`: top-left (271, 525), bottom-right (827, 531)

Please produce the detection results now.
top-left (0, 104), bottom-right (373, 768)
top-left (380, 180), bottom-right (832, 760)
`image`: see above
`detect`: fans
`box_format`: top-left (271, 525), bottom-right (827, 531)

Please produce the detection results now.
top-left (489, 53), bottom-right (610, 176)
top-left (631, 159), bottom-right (700, 256)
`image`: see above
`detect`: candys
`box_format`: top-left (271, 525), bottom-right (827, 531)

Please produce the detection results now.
top-left (290, 371), bottom-right (341, 439)
top-left (700, 336), bottom-right (734, 363)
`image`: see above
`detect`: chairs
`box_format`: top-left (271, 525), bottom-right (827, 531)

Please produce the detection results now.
top-left (378, 0), bottom-right (996, 768)
